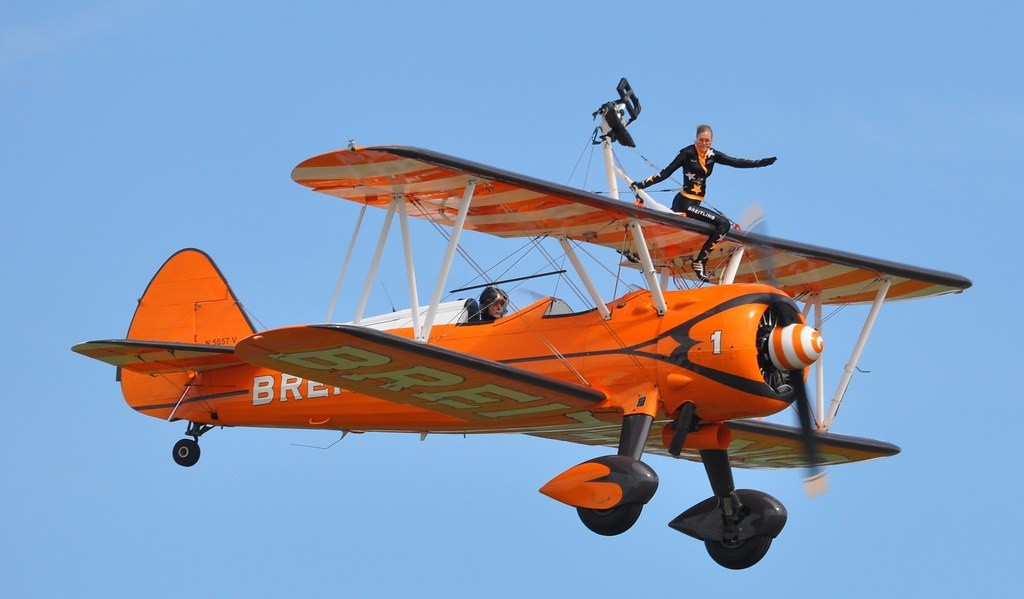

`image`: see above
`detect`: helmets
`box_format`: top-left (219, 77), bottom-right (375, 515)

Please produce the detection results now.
top-left (480, 287), bottom-right (508, 320)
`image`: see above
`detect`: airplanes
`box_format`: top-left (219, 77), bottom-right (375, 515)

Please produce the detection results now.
top-left (71, 78), bottom-right (973, 570)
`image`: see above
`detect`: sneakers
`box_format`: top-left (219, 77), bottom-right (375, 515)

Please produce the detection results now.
top-left (616, 248), bottom-right (640, 264)
top-left (691, 259), bottom-right (710, 282)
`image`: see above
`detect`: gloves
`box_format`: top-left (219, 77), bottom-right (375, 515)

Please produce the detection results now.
top-left (629, 182), bottom-right (645, 190)
top-left (756, 157), bottom-right (777, 167)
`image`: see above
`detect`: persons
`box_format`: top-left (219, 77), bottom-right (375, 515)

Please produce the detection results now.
top-left (617, 124), bottom-right (777, 284)
top-left (479, 288), bottom-right (509, 322)
